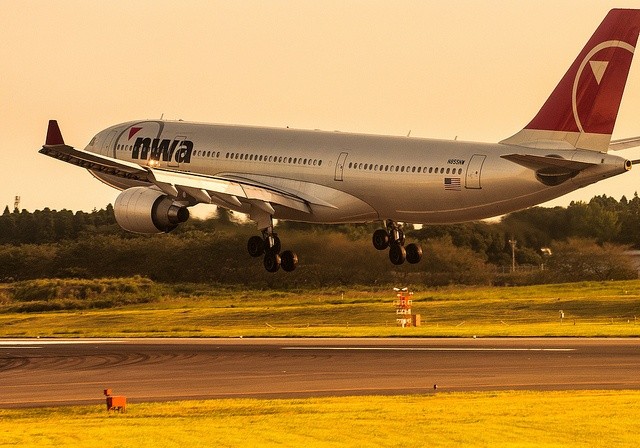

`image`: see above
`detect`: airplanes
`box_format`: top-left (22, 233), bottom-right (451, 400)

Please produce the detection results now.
top-left (38, 8), bottom-right (640, 272)
